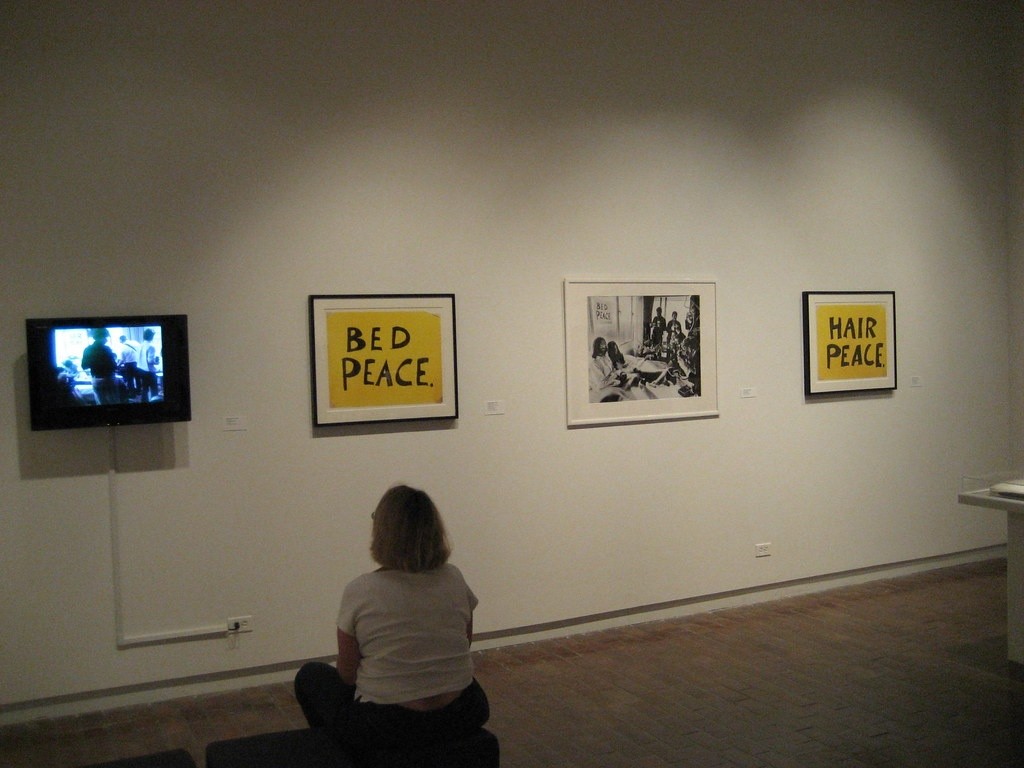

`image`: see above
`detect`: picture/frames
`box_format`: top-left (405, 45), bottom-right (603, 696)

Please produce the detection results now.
top-left (307, 295), bottom-right (458, 428)
top-left (801, 290), bottom-right (897, 396)
top-left (562, 278), bottom-right (720, 428)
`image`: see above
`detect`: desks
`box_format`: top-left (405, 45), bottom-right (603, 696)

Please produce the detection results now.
top-left (959, 489), bottom-right (1024, 679)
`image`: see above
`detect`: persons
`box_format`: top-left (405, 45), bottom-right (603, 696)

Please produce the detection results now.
top-left (52, 326), bottom-right (159, 405)
top-left (294, 483), bottom-right (489, 748)
top-left (590, 305), bottom-right (705, 402)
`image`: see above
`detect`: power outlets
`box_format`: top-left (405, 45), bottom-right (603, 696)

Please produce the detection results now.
top-left (755, 543), bottom-right (772, 557)
top-left (227, 616), bottom-right (253, 635)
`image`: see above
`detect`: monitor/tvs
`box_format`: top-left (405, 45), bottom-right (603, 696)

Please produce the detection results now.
top-left (26, 314), bottom-right (192, 430)
